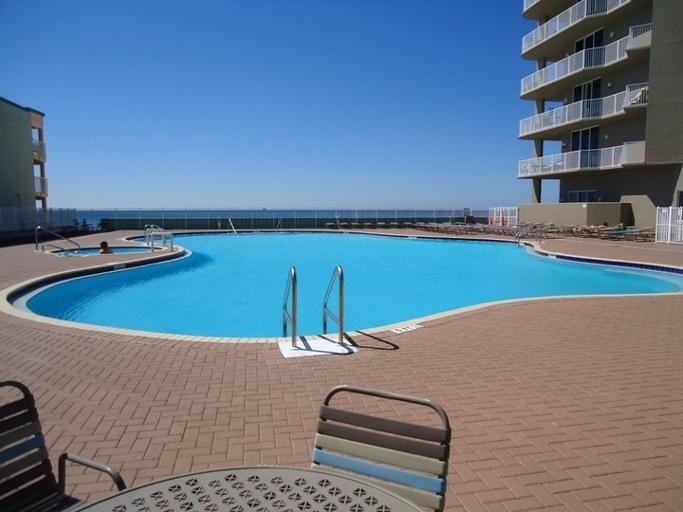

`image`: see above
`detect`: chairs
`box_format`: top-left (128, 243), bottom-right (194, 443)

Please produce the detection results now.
top-left (0, 380), bottom-right (127, 511)
top-left (311, 384), bottom-right (451, 512)
top-left (411, 221), bottom-right (656, 242)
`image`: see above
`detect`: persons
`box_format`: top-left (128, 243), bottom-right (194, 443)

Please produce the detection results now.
top-left (596, 222), bottom-right (607, 227)
top-left (98, 241), bottom-right (114, 254)
top-left (601, 223), bottom-right (626, 231)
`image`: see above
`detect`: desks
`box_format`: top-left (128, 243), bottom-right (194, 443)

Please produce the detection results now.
top-left (63, 464), bottom-right (426, 512)
